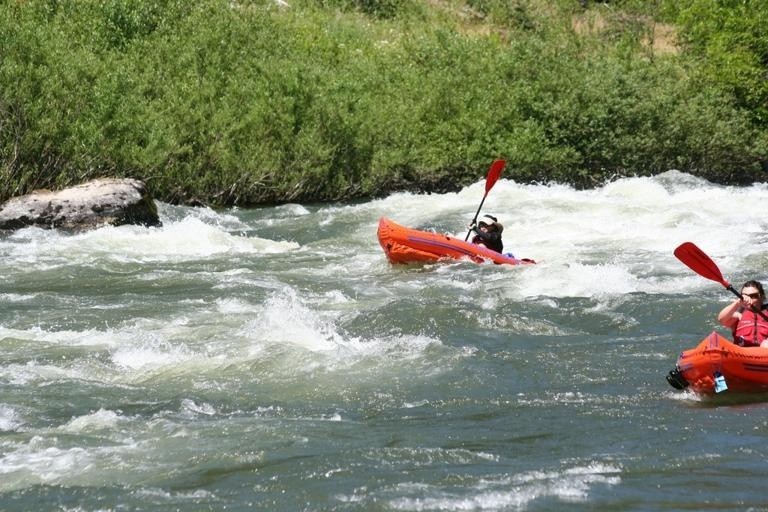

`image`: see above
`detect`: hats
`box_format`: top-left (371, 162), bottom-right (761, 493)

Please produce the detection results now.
top-left (477, 216), bottom-right (496, 227)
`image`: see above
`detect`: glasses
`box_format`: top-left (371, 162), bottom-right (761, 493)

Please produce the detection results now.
top-left (746, 293), bottom-right (761, 299)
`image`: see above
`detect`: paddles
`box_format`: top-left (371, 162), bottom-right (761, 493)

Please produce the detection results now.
top-left (465, 160), bottom-right (505, 243)
top-left (674, 243), bottom-right (768, 322)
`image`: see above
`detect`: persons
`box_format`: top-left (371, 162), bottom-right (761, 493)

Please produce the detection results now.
top-left (466, 214), bottom-right (504, 253)
top-left (717, 280), bottom-right (768, 348)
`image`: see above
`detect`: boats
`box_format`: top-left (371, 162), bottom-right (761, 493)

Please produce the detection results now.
top-left (666, 330), bottom-right (768, 397)
top-left (376, 214), bottom-right (536, 265)
